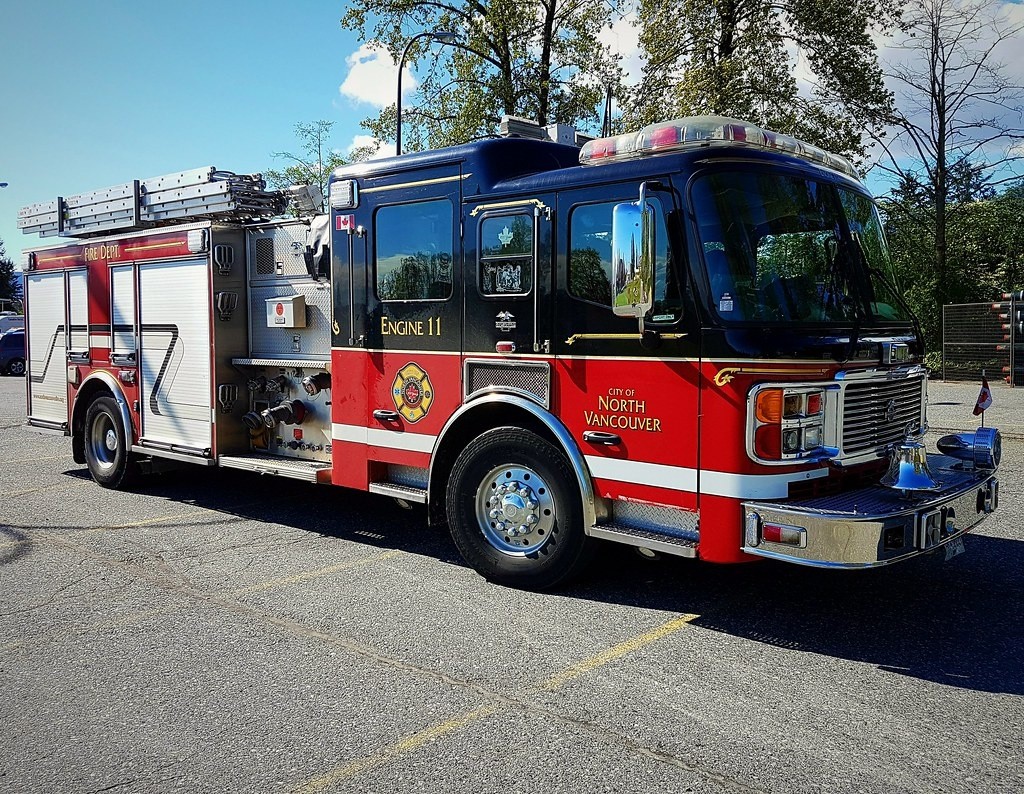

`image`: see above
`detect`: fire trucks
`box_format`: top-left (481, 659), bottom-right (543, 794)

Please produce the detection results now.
top-left (15, 115), bottom-right (1001, 596)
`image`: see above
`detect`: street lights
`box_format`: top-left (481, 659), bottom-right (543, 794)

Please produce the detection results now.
top-left (396, 31), bottom-right (460, 155)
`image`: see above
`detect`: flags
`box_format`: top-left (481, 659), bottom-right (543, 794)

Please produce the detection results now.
top-left (972, 374), bottom-right (993, 415)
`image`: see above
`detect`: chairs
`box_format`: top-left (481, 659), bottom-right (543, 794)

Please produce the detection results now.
top-left (705, 249), bottom-right (756, 306)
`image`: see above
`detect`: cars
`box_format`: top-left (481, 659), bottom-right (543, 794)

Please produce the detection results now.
top-left (0, 311), bottom-right (26, 376)
